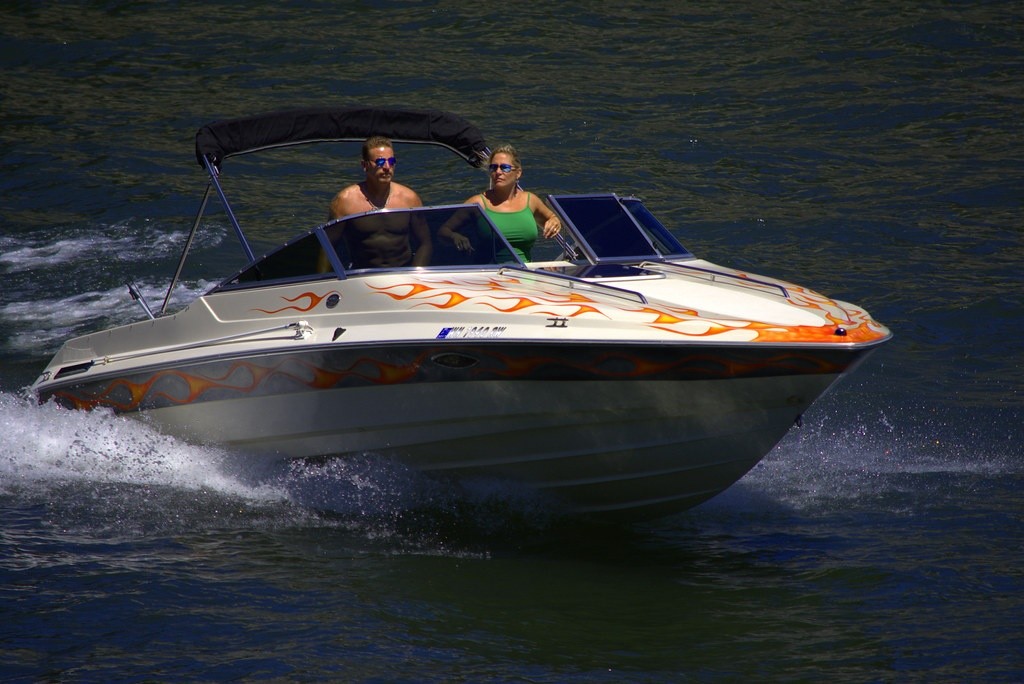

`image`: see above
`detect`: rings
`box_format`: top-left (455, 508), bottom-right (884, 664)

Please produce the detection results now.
top-left (554, 228), bottom-right (557, 232)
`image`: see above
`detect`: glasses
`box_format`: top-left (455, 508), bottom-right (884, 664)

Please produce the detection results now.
top-left (489, 163), bottom-right (516, 173)
top-left (369, 157), bottom-right (397, 166)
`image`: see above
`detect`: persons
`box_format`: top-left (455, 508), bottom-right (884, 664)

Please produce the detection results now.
top-left (437, 143), bottom-right (561, 264)
top-left (317, 135), bottom-right (435, 275)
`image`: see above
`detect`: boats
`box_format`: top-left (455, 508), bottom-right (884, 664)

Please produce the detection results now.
top-left (25, 104), bottom-right (896, 531)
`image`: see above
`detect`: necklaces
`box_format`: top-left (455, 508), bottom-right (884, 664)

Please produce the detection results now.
top-left (365, 180), bottom-right (391, 210)
